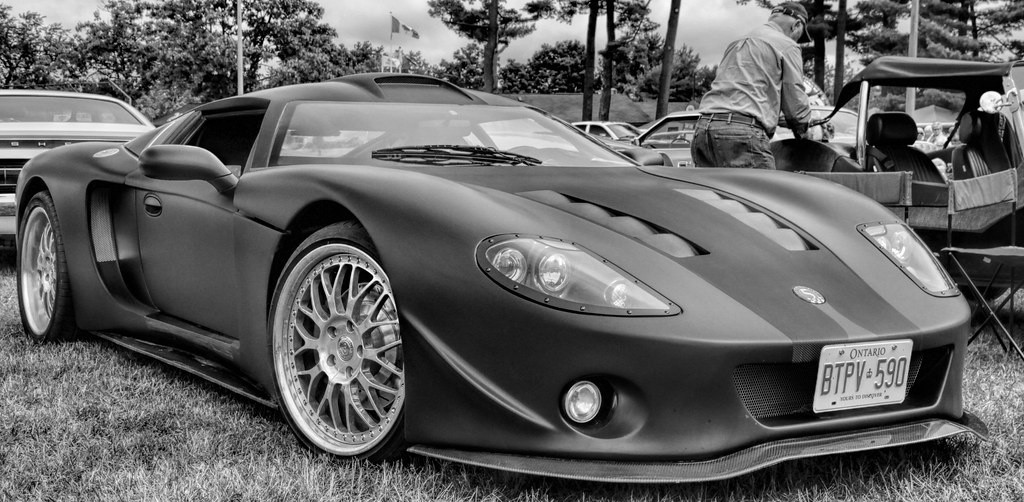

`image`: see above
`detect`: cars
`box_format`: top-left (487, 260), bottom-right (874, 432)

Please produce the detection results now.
top-left (1, 90), bottom-right (159, 248)
top-left (572, 101), bottom-right (870, 169)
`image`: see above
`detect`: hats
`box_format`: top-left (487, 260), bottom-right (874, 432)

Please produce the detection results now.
top-left (771, 1), bottom-right (813, 44)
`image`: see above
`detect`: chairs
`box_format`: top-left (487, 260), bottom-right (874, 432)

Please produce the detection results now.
top-left (866, 113), bottom-right (944, 184)
top-left (390, 126), bottom-right (469, 148)
top-left (941, 246), bottom-right (1024, 361)
top-left (952, 109), bottom-right (1023, 179)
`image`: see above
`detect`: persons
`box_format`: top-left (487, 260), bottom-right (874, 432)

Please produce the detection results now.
top-left (691, 1), bottom-right (814, 175)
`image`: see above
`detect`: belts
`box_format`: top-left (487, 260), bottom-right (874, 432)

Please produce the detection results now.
top-left (701, 113), bottom-right (766, 131)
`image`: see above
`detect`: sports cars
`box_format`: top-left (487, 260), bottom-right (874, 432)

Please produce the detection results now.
top-left (14, 72), bottom-right (973, 483)
